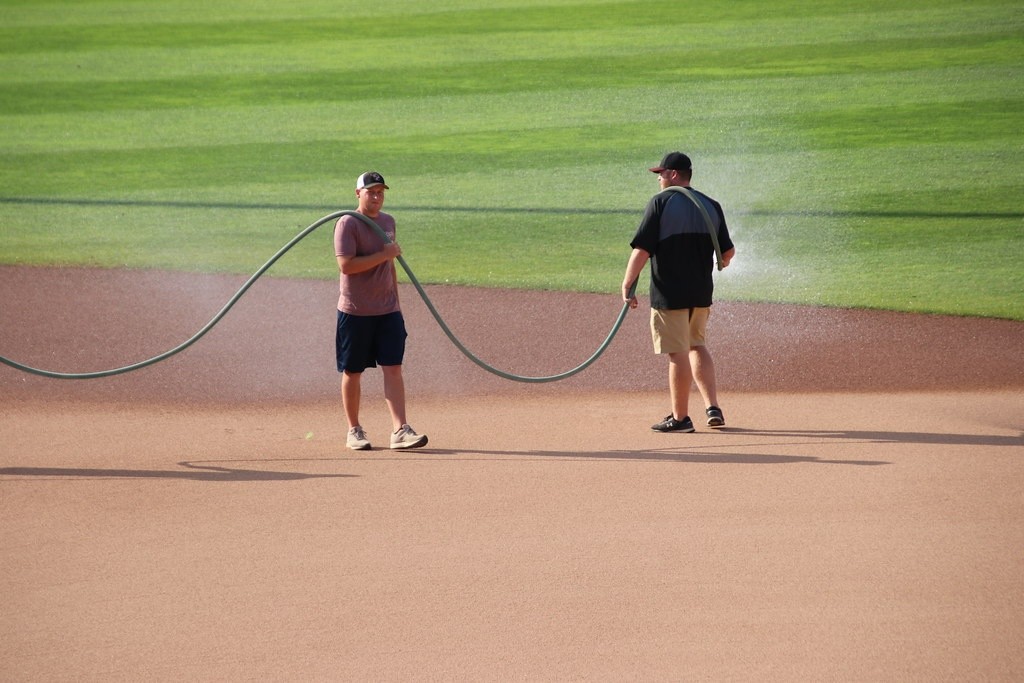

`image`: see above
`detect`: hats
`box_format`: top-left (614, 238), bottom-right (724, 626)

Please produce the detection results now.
top-left (357, 172), bottom-right (389, 191)
top-left (649, 151), bottom-right (691, 174)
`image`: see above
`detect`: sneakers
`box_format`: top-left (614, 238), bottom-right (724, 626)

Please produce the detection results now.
top-left (707, 410), bottom-right (725, 428)
top-left (389, 425), bottom-right (428, 449)
top-left (651, 412), bottom-right (695, 433)
top-left (346, 426), bottom-right (371, 450)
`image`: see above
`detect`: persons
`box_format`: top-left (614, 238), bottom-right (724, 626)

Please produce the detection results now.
top-left (621, 151), bottom-right (736, 432)
top-left (335, 171), bottom-right (429, 450)
